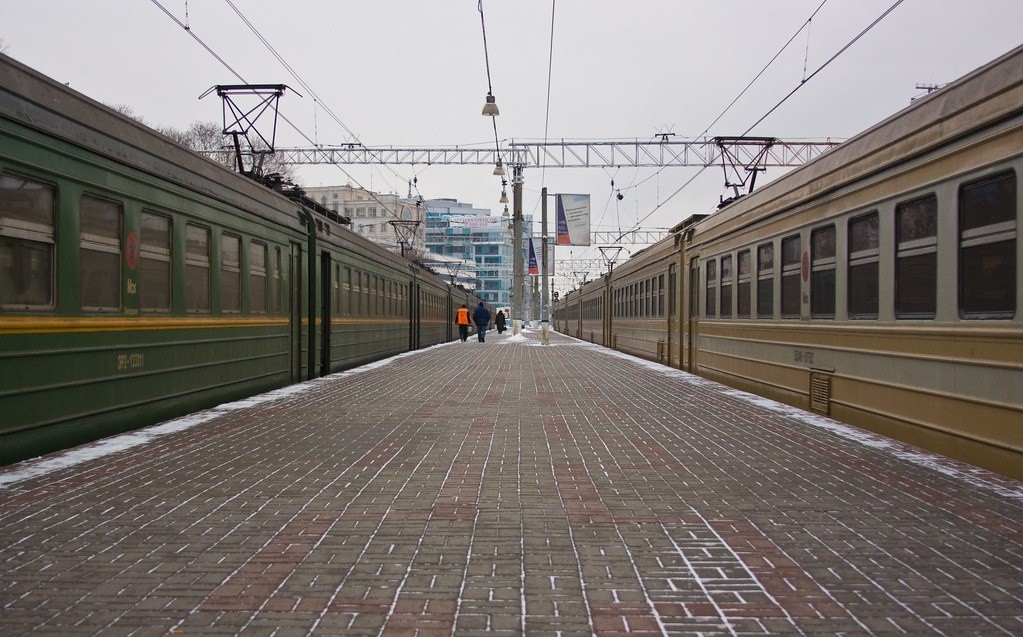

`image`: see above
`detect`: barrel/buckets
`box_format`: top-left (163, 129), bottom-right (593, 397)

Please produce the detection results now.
top-left (466, 325), bottom-right (473, 334)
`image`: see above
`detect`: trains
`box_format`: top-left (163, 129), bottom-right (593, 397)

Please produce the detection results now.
top-left (550, 44), bottom-right (1023, 480)
top-left (0, 55), bottom-right (498, 467)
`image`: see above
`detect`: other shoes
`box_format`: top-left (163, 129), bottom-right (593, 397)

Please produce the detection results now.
top-left (479, 337), bottom-right (485, 342)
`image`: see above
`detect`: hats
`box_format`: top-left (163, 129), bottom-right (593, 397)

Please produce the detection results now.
top-left (479, 302), bottom-right (484, 306)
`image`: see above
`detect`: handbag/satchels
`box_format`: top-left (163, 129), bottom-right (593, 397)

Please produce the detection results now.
top-left (467, 322), bottom-right (473, 334)
top-left (502, 325), bottom-right (507, 330)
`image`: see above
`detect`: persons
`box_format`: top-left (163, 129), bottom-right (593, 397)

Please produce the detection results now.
top-left (495, 311), bottom-right (505, 333)
top-left (455, 304), bottom-right (471, 341)
top-left (473, 302), bottom-right (490, 342)
